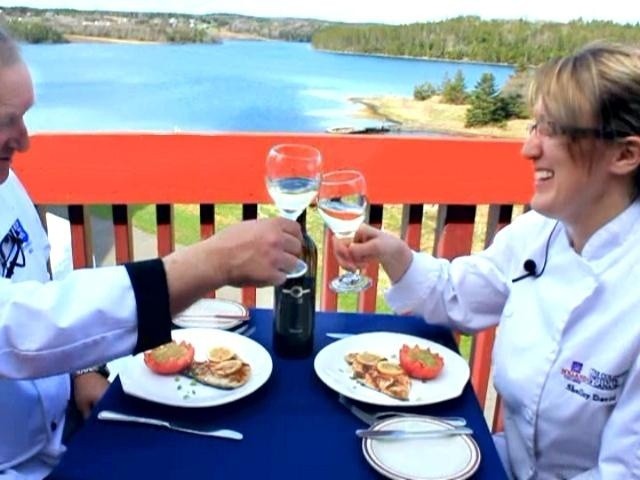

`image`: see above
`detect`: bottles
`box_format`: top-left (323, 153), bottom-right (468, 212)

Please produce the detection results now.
top-left (272, 206), bottom-right (316, 360)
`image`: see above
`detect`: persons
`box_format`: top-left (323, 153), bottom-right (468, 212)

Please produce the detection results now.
top-left (330, 38), bottom-right (639, 480)
top-left (0, 32), bottom-right (305, 479)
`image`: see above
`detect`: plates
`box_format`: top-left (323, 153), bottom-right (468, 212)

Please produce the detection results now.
top-left (172, 298), bottom-right (249, 327)
top-left (313, 331), bottom-right (472, 406)
top-left (108, 328), bottom-right (273, 408)
top-left (361, 417), bottom-right (482, 479)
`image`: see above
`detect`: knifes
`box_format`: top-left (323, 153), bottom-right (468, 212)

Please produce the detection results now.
top-left (96, 410), bottom-right (244, 441)
top-left (356, 428), bottom-right (474, 442)
top-left (179, 314), bottom-right (252, 322)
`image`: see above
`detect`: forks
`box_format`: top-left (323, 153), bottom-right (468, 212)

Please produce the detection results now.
top-left (338, 394), bottom-right (468, 426)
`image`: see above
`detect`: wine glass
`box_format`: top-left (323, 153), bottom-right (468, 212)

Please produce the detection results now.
top-left (316, 170), bottom-right (373, 294)
top-left (264, 144), bottom-right (322, 278)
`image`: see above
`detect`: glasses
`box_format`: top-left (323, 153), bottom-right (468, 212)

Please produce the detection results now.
top-left (526, 120), bottom-right (619, 141)
